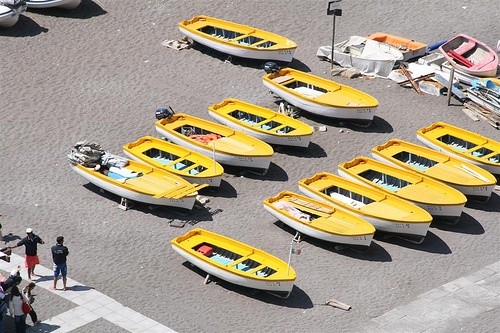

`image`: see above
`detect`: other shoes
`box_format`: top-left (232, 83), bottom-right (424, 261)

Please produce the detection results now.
top-left (34, 320), bottom-right (41, 325)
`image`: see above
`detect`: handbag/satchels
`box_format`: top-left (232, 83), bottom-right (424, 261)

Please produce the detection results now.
top-left (19, 293), bottom-right (32, 314)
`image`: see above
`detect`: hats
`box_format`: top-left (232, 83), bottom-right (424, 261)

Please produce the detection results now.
top-left (26, 228), bottom-right (33, 234)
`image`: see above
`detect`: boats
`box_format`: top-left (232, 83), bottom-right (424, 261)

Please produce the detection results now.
top-left (371, 139), bottom-right (496, 197)
top-left (298, 172), bottom-right (432, 236)
top-left (0, 1), bottom-right (27, 26)
top-left (264, 65), bottom-right (378, 118)
top-left (178, 11), bottom-right (295, 62)
top-left (437, 32), bottom-right (497, 71)
top-left (122, 130), bottom-right (222, 189)
top-left (417, 121), bottom-right (500, 173)
top-left (337, 157), bottom-right (467, 216)
top-left (27, 2), bottom-right (80, 12)
top-left (172, 228), bottom-right (295, 292)
top-left (265, 190), bottom-right (374, 245)
top-left (67, 141), bottom-right (198, 210)
top-left (156, 99), bottom-right (273, 172)
top-left (208, 96), bottom-right (313, 148)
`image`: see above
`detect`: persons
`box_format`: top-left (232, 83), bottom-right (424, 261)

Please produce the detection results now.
top-left (21, 282), bottom-right (41, 326)
top-left (0, 286), bottom-right (11, 322)
top-left (51, 236), bottom-right (70, 291)
top-left (9, 286), bottom-right (30, 333)
top-left (9, 227), bottom-right (45, 280)
top-left (0, 265), bottom-right (22, 293)
